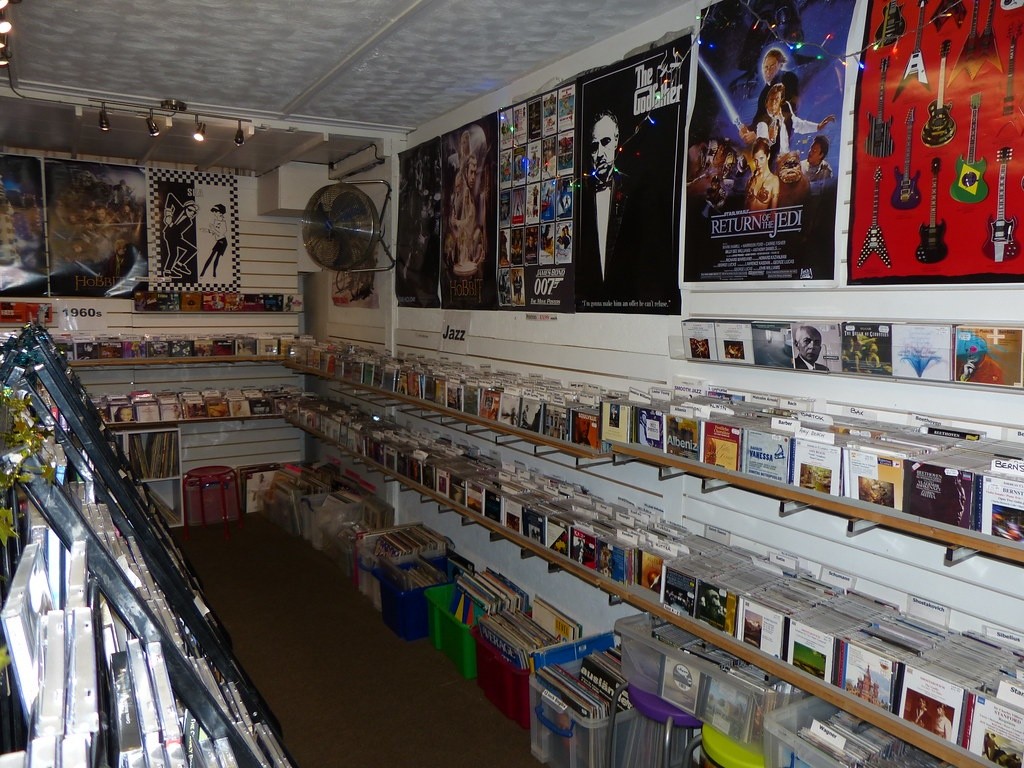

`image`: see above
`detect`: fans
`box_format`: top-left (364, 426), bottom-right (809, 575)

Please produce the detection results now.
top-left (300, 180), bottom-right (382, 272)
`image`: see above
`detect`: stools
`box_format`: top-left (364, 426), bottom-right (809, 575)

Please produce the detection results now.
top-left (683, 722), bottom-right (764, 768)
top-left (603, 681), bottom-right (703, 768)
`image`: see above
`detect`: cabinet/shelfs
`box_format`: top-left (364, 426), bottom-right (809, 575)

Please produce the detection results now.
top-left (110, 425), bottom-right (185, 528)
top-left (281, 359), bottom-right (1024, 768)
top-left (68, 357), bottom-right (288, 432)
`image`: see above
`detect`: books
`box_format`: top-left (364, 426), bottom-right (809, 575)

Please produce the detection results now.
top-left (0, 333), bottom-right (278, 768)
top-left (273, 331), bottom-right (1023, 768)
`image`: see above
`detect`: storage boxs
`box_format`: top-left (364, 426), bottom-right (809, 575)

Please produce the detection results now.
top-left (263, 472), bottom-right (856, 768)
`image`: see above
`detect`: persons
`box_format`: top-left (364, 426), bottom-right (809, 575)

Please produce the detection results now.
top-left (909, 697), bottom-right (932, 730)
top-left (933, 704), bottom-right (951, 741)
top-left (794, 326), bottom-right (830, 371)
top-left (575, 110), bottom-right (657, 301)
top-left (689, 49), bottom-right (833, 216)
top-left (55, 173), bottom-right (145, 277)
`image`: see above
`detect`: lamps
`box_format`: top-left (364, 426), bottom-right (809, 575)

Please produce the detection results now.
top-left (88, 98), bottom-right (254, 148)
